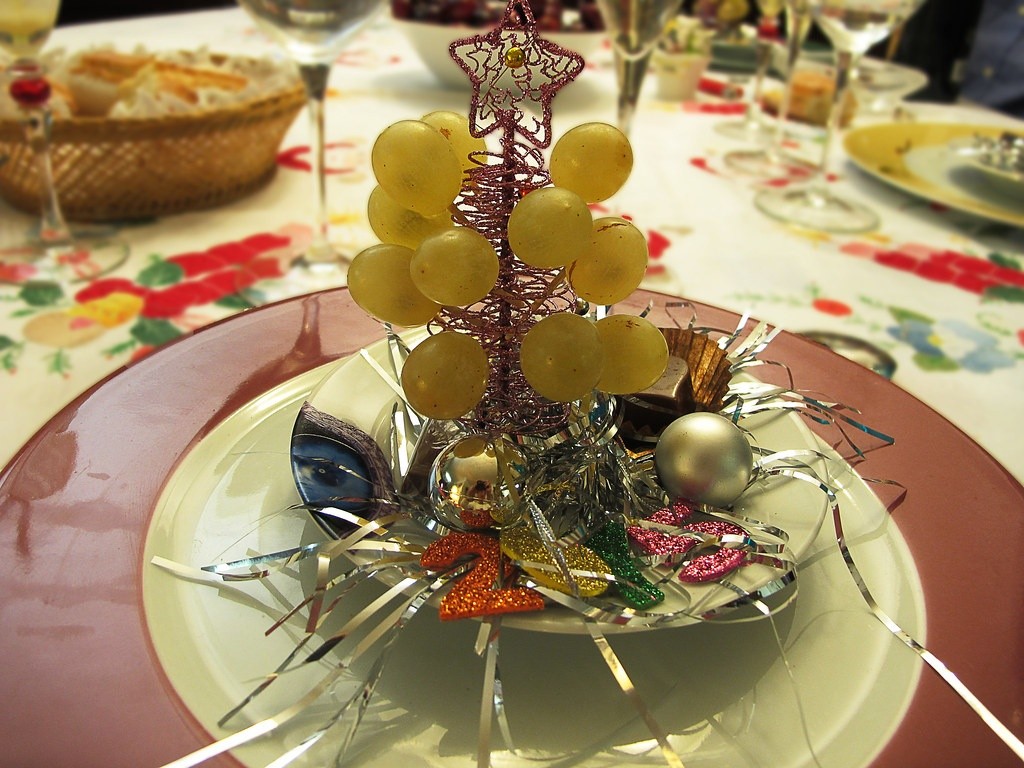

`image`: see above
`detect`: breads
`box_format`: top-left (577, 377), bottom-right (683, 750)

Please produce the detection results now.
top-left (77, 51), bottom-right (248, 92)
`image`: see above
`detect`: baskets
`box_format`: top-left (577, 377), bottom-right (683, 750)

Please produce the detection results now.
top-left (0, 55), bottom-right (307, 219)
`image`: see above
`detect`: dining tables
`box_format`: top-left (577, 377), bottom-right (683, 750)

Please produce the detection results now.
top-left (0, 0), bottom-right (1024, 768)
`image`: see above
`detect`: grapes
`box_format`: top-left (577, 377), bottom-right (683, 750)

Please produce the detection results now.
top-left (343, 109), bottom-right (670, 420)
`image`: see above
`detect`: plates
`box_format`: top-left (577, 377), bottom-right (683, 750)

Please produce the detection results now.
top-left (0, 291), bottom-right (1024, 767)
top-left (961, 155), bottom-right (1024, 195)
top-left (841, 122), bottom-right (1024, 229)
top-left (777, 48), bottom-right (929, 97)
top-left (290, 319), bottom-right (825, 632)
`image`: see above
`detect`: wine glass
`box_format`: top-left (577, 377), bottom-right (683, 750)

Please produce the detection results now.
top-left (595, 0), bottom-right (684, 217)
top-left (234, 0), bottom-right (390, 308)
top-left (713, 0), bottom-right (929, 233)
top-left (0, 0), bottom-right (130, 287)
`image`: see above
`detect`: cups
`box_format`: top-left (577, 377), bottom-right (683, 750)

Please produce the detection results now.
top-left (654, 43), bottom-right (709, 100)
top-left (848, 61), bottom-right (908, 127)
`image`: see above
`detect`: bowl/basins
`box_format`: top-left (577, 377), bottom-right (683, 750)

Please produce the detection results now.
top-left (394, 20), bottom-right (614, 96)
